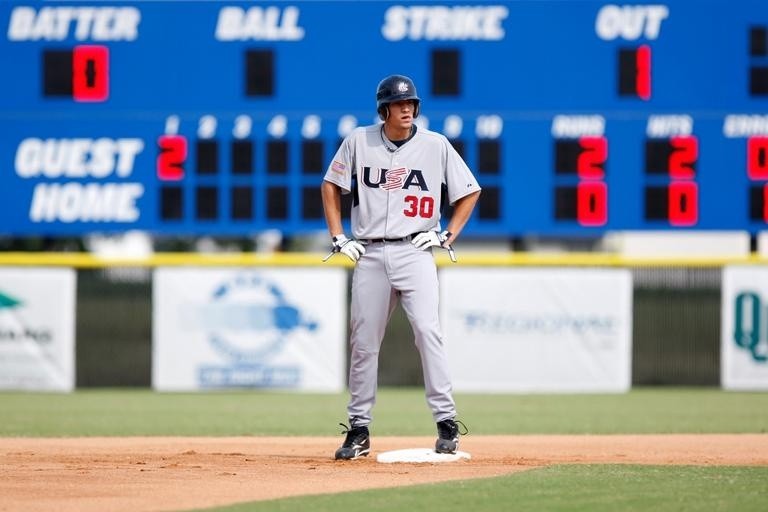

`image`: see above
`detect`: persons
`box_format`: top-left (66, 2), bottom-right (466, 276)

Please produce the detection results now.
top-left (319, 72), bottom-right (484, 460)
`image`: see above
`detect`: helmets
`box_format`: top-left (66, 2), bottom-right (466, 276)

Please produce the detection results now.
top-left (376, 76), bottom-right (420, 109)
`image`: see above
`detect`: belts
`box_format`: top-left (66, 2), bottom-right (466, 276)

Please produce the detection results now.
top-left (358, 232), bottom-right (429, 243)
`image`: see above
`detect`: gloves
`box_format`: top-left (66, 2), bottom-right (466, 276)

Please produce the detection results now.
top-left (335, 233), bottom-right (369, 264)
top-left (410, 229), bottom-right (457, 263)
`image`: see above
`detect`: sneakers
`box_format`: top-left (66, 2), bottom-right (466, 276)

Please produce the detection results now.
top-left (335, 422), bottom-right (372, 460)
top-left (435, 419), bottom-right (469, 454)
